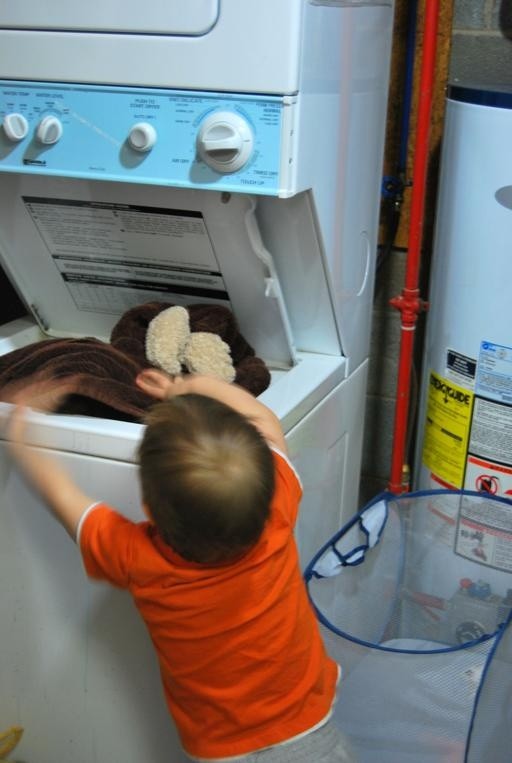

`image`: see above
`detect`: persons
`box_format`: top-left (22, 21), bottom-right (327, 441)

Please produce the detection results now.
top-left (3, 368), bottom-right (354, 763)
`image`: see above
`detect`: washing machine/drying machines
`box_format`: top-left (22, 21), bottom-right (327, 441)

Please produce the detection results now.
top-left (0, 0), bottom-right (397, 763)
